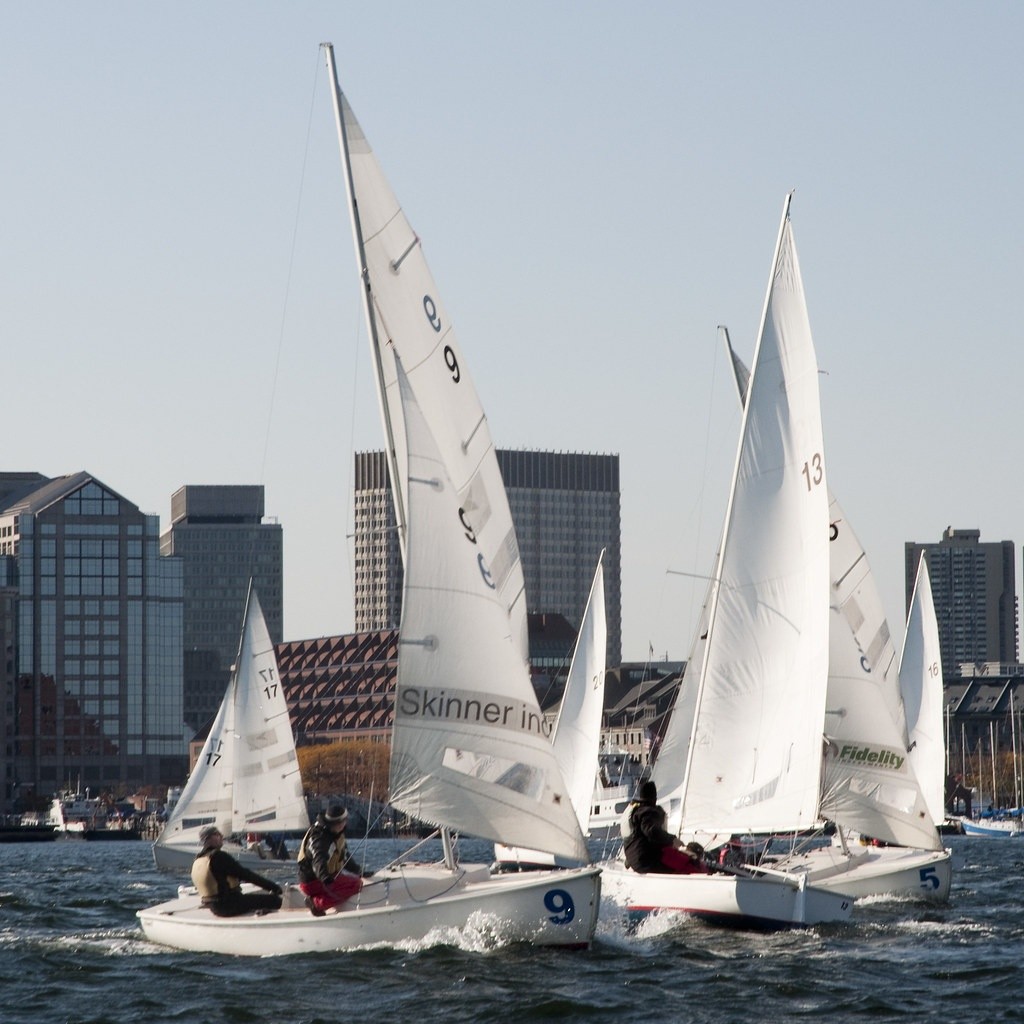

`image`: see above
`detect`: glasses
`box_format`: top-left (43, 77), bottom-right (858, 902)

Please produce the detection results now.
top-left (209, 832), bottom-right (221, 837)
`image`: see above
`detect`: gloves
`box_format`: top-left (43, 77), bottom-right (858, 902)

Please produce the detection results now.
top-left (324, 877), bottom-right (334, 888)
top-left (273, 884), bottom-right (283, 894)
top-left (360, 869), bottom-right (374, 878)
top-left (673, 838), bottom-right (685, 849)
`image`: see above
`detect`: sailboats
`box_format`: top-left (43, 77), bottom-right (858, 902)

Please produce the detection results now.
top-left (0, 36), bottom-right (1024, 963)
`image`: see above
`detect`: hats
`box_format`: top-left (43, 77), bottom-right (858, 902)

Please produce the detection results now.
top-left (325, 805), bottom-right (352, 824)
top-left (639, 782), bottom-right (657, 800)
top-left (200, 824), bottom-right (216, 846)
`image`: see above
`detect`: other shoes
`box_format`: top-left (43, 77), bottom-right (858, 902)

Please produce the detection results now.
top-left (304, 896), bottom-right (326, 918)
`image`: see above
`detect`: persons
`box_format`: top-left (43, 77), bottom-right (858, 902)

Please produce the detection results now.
top-left (231, 832), bottom-right (242, 846)
top-left (266, 831), bottom-right (282, 852)
top-left (625, 782), bottom-right (709, 875)
top-left (247, 831), bottom-right (262, 851)
top-left (297, 805), bottom-right (374, 917)
top-left (191, 826), bottom-right (282, 917)
top-left (972, 808), bottom-right (981, 820)
top-left (720, 834), bottom-right (745, 868)
